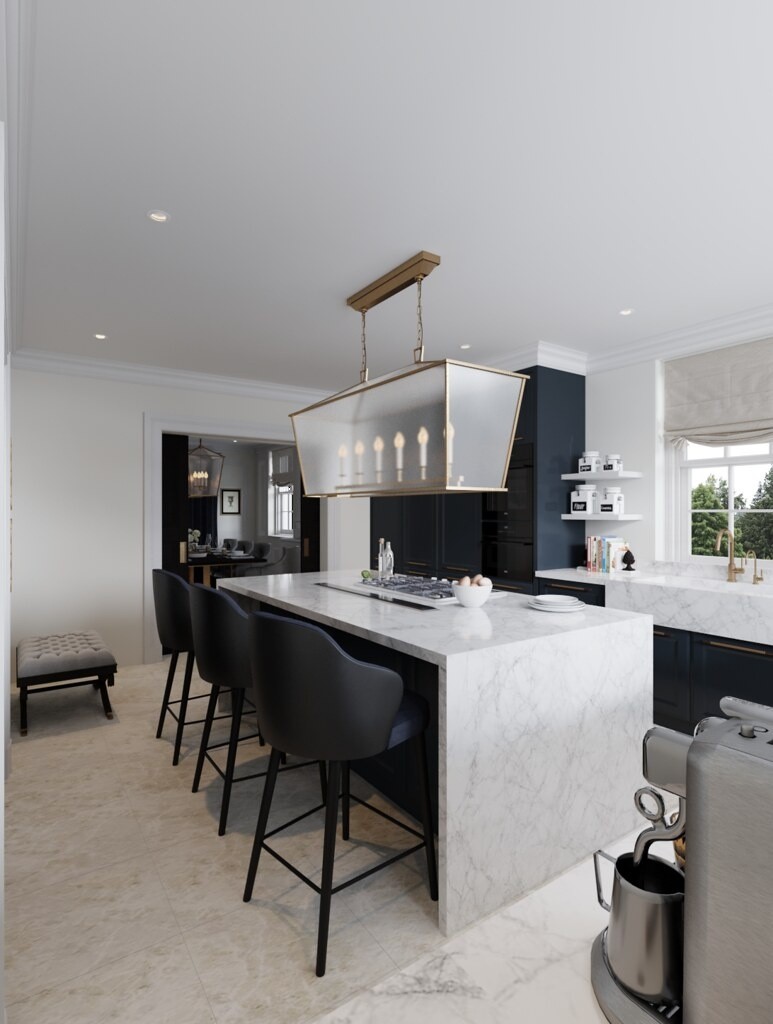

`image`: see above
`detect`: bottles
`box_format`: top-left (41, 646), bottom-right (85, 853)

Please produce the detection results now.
top-left (382, 542), bottom-right (394, 577)
top-left (378, 538), bottom-right (385, 575)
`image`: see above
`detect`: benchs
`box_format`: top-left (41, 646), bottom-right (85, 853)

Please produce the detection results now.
top-left (14, 630), bottom-right (117, 737)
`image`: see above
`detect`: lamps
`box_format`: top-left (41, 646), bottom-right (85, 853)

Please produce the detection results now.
top-left (188, 438), bottom-right (225, 497)
top-left (288, 250), bottom-right (531, 498)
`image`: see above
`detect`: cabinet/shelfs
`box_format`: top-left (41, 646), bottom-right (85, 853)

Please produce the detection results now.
top-left (272, 447), bottom-right (297, 488)
top-left (653, 624), bottom-right (691, 735)
top-left (369, 366), bottom-right (587, 593)
top-left (691, 633), bottom-right (773, 736)
top-left (561, 451), bottom-right (643, 521)
top-left (300, 468), bottom-right (320, 574)
top-left (538, 578), bottom-right (606, 608)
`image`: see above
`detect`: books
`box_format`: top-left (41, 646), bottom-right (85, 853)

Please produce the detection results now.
top-left (587, 535), bottom-right (629, 574)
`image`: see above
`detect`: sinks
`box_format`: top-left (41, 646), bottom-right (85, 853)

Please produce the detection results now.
top-left (639, 576), bottom-right (773, 592)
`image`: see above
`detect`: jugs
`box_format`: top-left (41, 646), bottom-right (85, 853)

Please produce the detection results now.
top-left (594, 849), bottom-right (685, 1005)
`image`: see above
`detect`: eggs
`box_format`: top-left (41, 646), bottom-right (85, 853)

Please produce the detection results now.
top-left (457, 574), bottom-right (492, 587)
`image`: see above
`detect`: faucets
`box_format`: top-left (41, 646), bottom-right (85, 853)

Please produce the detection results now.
top-left (746, 550), bottom-right (765, 585)
top-left (715, 528), bottom-right (746, 582)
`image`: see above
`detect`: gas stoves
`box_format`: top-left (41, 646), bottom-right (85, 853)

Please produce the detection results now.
top-left (356, 575), bottom-right (504, 602)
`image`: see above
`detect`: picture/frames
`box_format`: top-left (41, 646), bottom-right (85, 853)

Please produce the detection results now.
top-left (221, 488), bottom-right (241, 515)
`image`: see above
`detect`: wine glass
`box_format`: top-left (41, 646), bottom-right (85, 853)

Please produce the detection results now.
top-left (206, 534), bottom-right (211, 545)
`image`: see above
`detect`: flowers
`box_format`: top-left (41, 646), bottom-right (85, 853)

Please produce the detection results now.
top-left (188, 529), bottom-right (201, 550)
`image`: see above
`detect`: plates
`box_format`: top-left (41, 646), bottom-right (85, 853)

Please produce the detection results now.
top-left (527, 595), bottom-right (585, 611)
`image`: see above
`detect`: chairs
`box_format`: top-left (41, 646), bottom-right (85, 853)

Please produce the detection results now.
top-left (152, 569), bottom-right (265, 767)
top-left (241, 611), bottom-right (438, 978)
top-left (228, 539), bottom-right (253, 578)
top-left (189, 583), bottom-right (326, 836)
top-left (213, 539), bottom-right (237, 580)
top-left (250, 546), bottom-right (288, 576)
top-left (243, 542), bottom-right (273, 575)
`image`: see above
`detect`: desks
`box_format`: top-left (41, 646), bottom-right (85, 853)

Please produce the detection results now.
top-left (216, 568), bottom-right (654, 938)
top-left (188, 545), bottom-right (268, 588)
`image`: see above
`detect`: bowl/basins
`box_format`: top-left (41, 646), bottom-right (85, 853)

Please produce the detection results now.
top-left (452, 583), bottom-right (493, 607)
